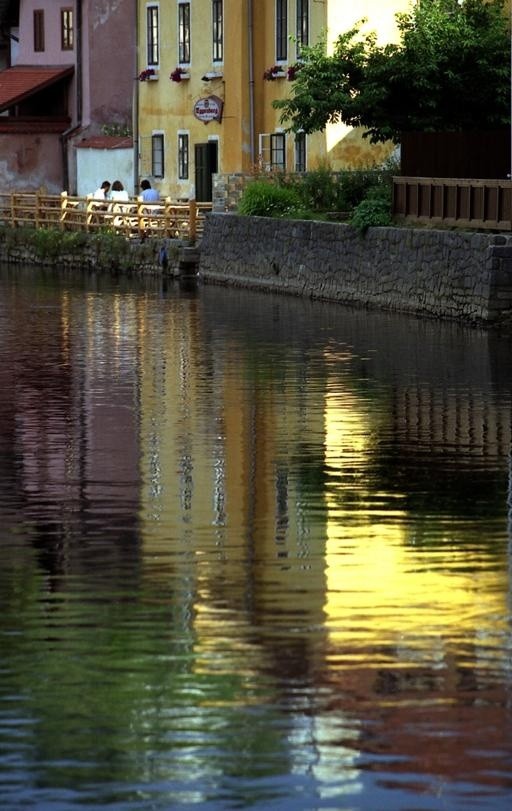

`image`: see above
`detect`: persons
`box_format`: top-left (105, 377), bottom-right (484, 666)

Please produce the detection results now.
top-left (138, 179), bottom-right (160, 215)
top-left (107, 179), bottom-right (129, 214)
top-left (92, 180), bottom-right (110, 210)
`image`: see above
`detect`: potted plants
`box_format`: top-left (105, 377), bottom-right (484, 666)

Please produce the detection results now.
top-left (169, 67), bottom-right (190, 83)
top-left (263, 64), bottom-right (286, 82)
top-left (134, 68), bottom-right (159, 81)
